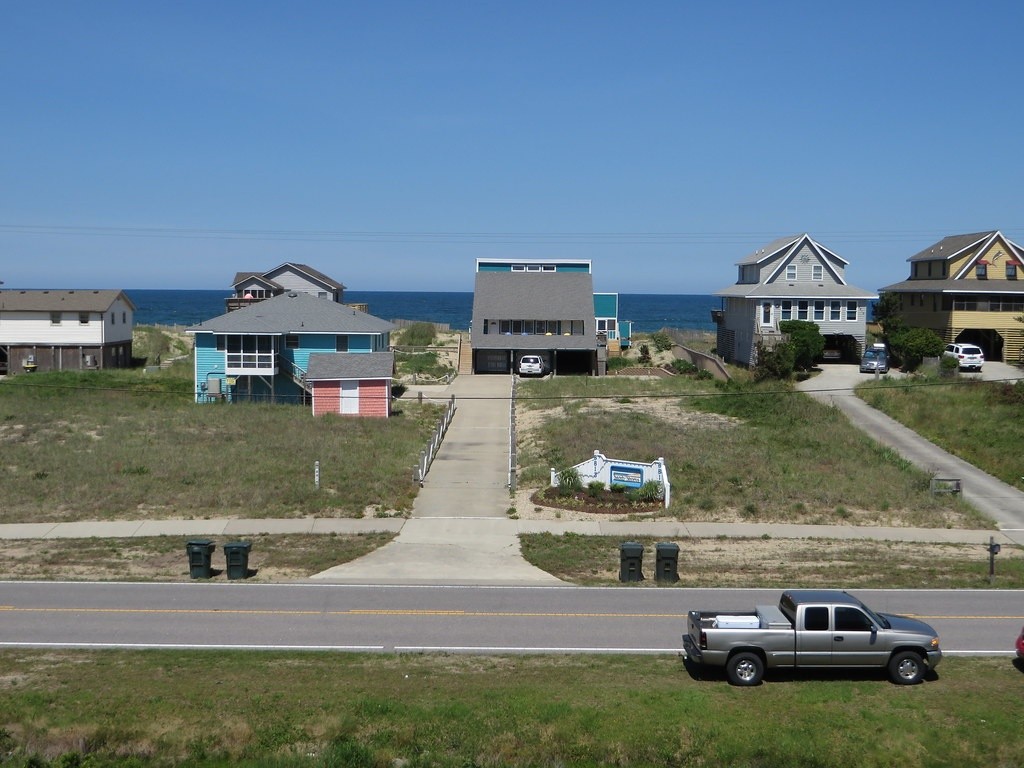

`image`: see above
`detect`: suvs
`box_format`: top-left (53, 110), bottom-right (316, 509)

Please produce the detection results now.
top-left (944, 344), bottom-right (984, 371)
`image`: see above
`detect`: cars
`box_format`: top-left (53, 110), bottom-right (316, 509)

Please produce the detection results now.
top-left (519, 356), bottom-right (546, 377)
top-left (820, 345), bottom-right (841, 363)
top-left (1015, 624), bottom-right (1024, 660)
top-left (0, 346), bottom-right (8, 375)
top-left (872, 343), bottom-right (886, 351)
top-left (861, 348), bottom-right (890, 373)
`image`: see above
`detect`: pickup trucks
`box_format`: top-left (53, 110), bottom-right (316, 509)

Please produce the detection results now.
top-left (682, 590), bottom-right (943, 686)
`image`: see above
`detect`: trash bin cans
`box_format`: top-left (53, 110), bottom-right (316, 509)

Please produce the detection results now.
top-left (185, 539), bottom-right (213, 579)
top-left (619, 543), bottom-right (645, 583)
top-left (654, 543), bottom-right (679, 583)
top-left (222, 542), bottom-right (252, 580)
